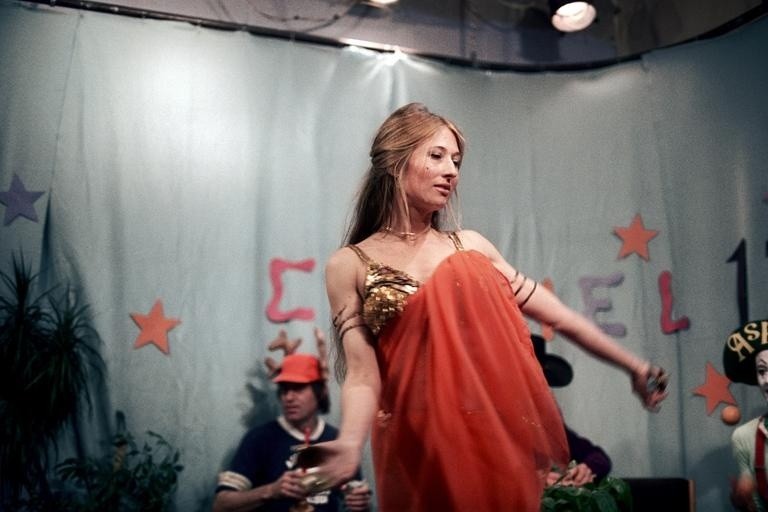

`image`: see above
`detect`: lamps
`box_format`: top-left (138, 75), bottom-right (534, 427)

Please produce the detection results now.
top-left (550, 0), bottom-right (596, 32)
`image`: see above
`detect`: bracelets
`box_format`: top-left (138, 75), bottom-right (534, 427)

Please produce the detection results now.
top-left (627, 356), bottom-right (648, 383)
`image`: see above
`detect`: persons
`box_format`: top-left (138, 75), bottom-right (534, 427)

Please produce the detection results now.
top-left (212, 351), bottom-right (374, 510)
top-left (528, 333), bottom-right (613, 512)
top-left (722, 317), bottom-right (768, 512)
top-left (307, 99), bottom-right (673, 512)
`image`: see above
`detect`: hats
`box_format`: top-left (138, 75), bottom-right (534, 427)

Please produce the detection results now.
top-left (722, 320), bottom-right (768, 385)
top-left (529, 334), bottom-right (572, 387)
top-left (272, 352), bottom-right (321, 385)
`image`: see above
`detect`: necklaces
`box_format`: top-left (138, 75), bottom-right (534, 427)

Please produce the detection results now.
top-left (377, 220), bottom-right (433, 243)
